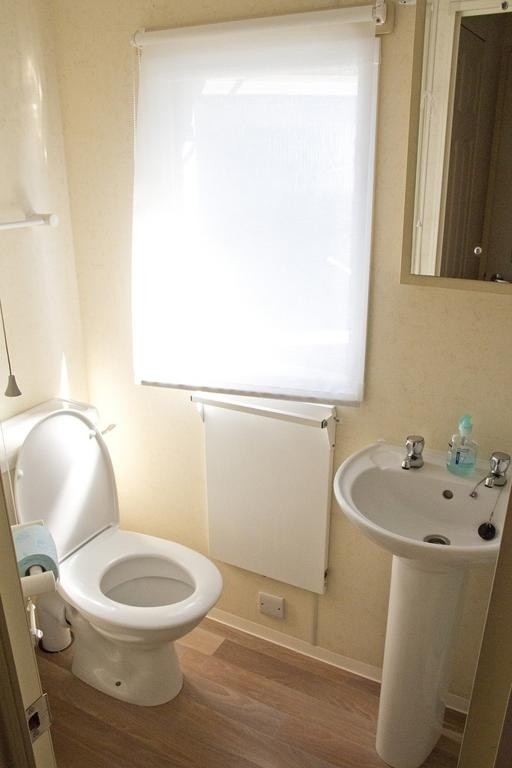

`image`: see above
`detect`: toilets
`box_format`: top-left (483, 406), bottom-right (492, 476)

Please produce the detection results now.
top-left (0, 398), bottom-right (223, 707)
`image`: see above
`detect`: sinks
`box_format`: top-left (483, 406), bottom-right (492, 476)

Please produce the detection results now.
top-left (332, 439), bottom-right (511, 567)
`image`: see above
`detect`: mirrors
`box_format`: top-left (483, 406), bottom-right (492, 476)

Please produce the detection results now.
top-left (400, 0), bottom-right (512, 296)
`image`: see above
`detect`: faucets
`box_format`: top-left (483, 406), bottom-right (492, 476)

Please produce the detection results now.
top-left (401, 435), bottom-right (424, 469)
top-left (483, 452), bottom-right (510, 487)
